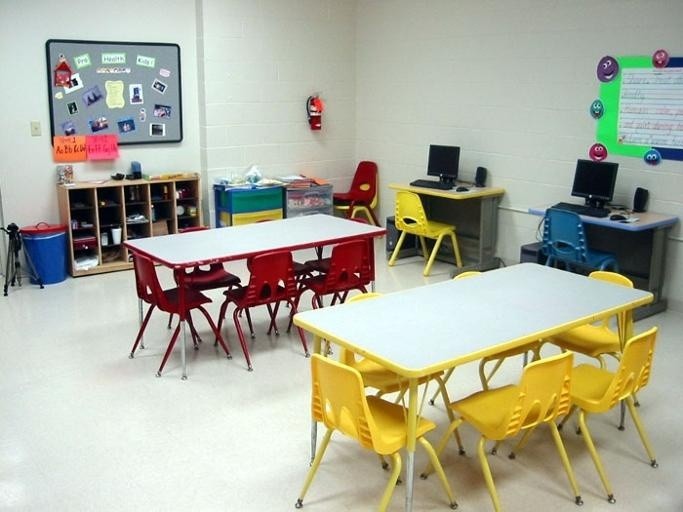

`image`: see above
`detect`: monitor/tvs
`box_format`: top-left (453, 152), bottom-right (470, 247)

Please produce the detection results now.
top-left (571, 159), bottom-right (619, 213)
top-left (427, 144), bottom-right (460, 187)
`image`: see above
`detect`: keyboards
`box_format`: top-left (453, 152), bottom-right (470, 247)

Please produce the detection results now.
top-left (410, 179), bottom-right (453, 190)
top-left (551, 202), bottom-right (608, 218)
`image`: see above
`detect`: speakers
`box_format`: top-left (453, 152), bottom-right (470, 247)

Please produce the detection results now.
top-left (633, 187), bottom-right (648, 212)
top-left (475, 167), bottom-right (487, 187)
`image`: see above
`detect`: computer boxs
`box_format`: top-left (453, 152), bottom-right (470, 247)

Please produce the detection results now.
top-left (387, 216), bottom-right (418, 260)
top-left (520, 241), bottom-right (566, 271)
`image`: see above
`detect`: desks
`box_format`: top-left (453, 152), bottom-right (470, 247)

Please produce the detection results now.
top-left (528, 201), bottom-right (678, 323)
top-left (290, 261), bottom-right (655, 511)
top-left (387, 184), bottom-right (505, 280)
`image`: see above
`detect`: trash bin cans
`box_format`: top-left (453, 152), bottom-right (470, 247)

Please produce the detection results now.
top-left (20, 221), bottom-right (68, 285)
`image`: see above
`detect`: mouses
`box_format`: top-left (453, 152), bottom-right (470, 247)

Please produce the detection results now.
top-left (610, 215), bottom-right (626, 220)
top-left (456, 186), bottom-right (469, 192)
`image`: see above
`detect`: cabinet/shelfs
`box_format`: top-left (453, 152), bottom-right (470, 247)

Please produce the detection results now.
top-left (58, 172), bottom-right (203, 278)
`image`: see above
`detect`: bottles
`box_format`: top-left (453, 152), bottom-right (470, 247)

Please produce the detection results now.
top-left (176, 189), bottom-right (187, 199)
top-left (130, 186), bottom-right (140, 201)
top-left (151, 205), bottom-right (156, 222)
top-left (101, 232), bottom-right (109, 246)
top-left (164, 185), bottom-right (168, 200)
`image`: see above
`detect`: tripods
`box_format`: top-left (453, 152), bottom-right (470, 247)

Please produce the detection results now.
top-left (0, 223), bottom-right (44, 296)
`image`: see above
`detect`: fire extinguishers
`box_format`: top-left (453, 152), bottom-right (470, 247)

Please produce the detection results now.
top-left (306, 96), bottom-right (321, 130)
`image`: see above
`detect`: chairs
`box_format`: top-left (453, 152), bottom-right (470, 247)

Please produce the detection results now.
top-left (427, 270), bottom-right (541, 462)
top-left (533, 268), bottom-right (636, 434)
top-left (508, 325), bottom-right (661, 505)
top-left (293, 352), bottom-right (459, 511)
top-left (334, 159), bottom-right (386, 241)
top-left (386, 189), bottom-right (462, 278)
top-left (543, 209), bottom-right (622, 277)
top-left (418, 351), bottom-right (585, 510)
top-left (336, 290), bottom-right (467, 473)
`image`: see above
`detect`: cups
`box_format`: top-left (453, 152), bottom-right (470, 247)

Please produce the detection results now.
top-left (112, 228), bottom-right (122, 245)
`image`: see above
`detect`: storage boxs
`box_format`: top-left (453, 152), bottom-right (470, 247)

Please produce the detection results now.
top-left (215, 185), bottom-right (335, 229)
